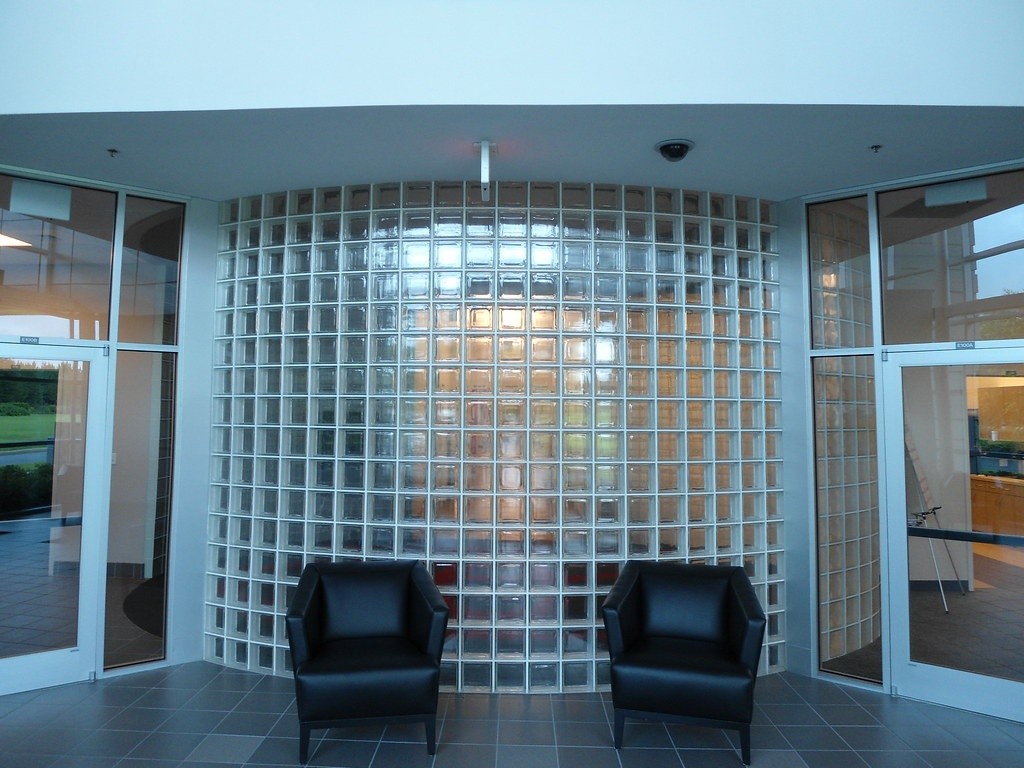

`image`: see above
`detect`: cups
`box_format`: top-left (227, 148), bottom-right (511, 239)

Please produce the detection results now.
top-left (992, 431), bottom-right (998, 440)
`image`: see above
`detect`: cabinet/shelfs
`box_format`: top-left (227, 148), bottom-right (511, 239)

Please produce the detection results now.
top-left (970, 474), bottom-right (1024, 536)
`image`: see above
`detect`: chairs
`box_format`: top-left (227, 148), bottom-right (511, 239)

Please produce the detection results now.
top-left (284, 561), bottom-right (450, 764)
top-left (602, 560), bottom-right (766, 765)
